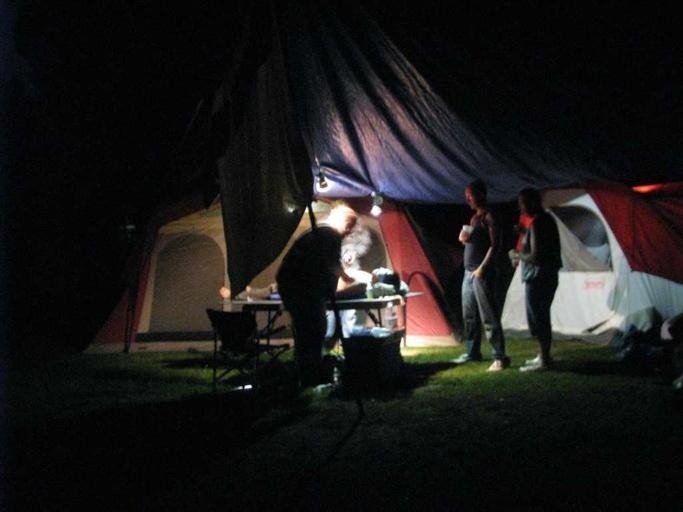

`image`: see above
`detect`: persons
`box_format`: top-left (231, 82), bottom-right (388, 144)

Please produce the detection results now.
top-left (270, 203), bottom-right (358, 383)
top-left (507, 185), bottom-right (565, 370)
top-left (449, 170), bottom-right (519, 374)
top-left (334, 218), bottom-right (386, 332)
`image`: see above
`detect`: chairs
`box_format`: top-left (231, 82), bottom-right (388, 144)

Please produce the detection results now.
top-left (202, 309), bottom-right (291, 395)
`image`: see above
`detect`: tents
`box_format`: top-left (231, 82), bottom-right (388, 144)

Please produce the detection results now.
top-left (74, 152), bottom-right (466, 359)
top-left (454, 176), bottom-right (681, 347)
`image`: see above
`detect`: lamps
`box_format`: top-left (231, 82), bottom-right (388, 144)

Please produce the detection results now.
top-left (370, 190), bottom-right (383, 217)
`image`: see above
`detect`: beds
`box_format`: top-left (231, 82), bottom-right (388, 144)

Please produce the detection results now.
top-left (218, 291), bottom-right (424, 353)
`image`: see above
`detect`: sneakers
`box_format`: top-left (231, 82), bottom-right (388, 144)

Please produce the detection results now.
top-left (449, 353), bottom-right (484, 366)
top-left (484, 357), bottom-right (510, 373)
top-left (518, 359), bottom-right (552, 374)
top-left (525, 353), bottom-right (543, 366)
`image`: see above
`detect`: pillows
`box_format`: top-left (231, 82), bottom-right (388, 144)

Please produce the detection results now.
top-left (371, 280), bottom-right (409, 298)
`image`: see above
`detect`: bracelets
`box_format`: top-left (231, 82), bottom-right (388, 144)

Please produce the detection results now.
top-left (514, 251), bottom-right (519, 258)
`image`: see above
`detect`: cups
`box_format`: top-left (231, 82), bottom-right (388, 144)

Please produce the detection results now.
top-left (463, 224), bottom-right (472, 239)
